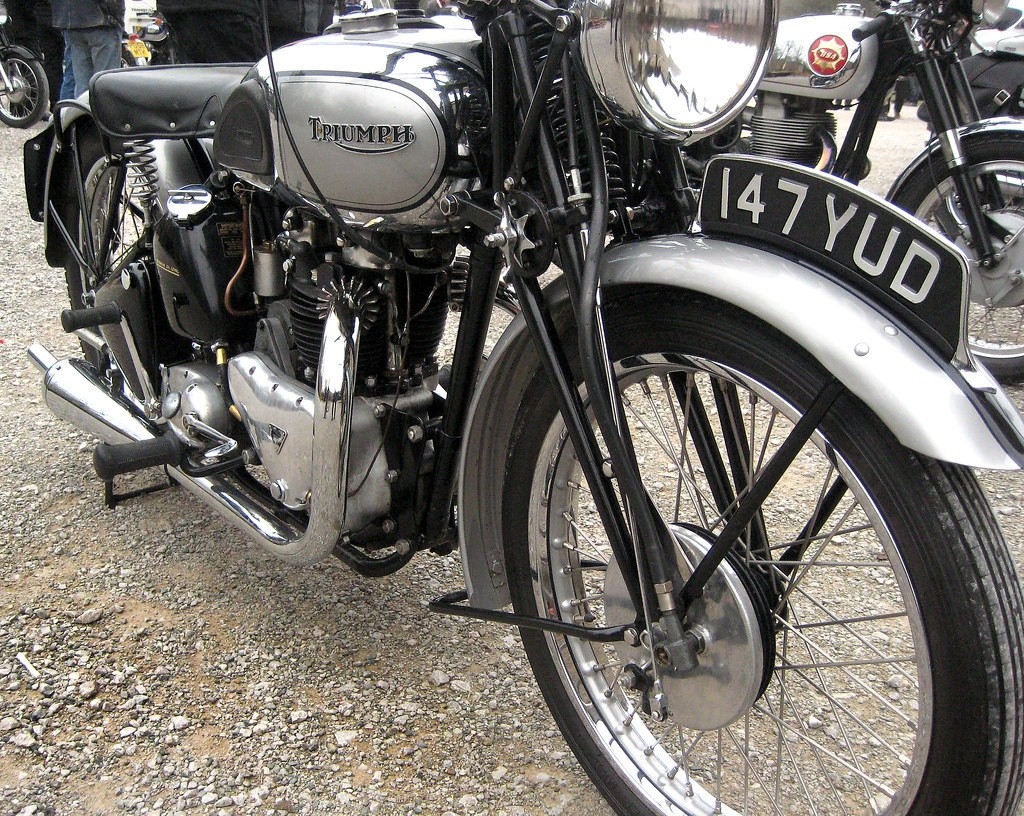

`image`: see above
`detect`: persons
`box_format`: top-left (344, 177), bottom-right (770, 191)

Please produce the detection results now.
top-left (154, 0), bottom-right (339, 64)
top-left (0, 0), bottom-right (125, 125)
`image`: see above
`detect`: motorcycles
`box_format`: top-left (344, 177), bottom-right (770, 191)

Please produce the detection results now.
top-left (0, 0), bottom-right (1024, 815)
